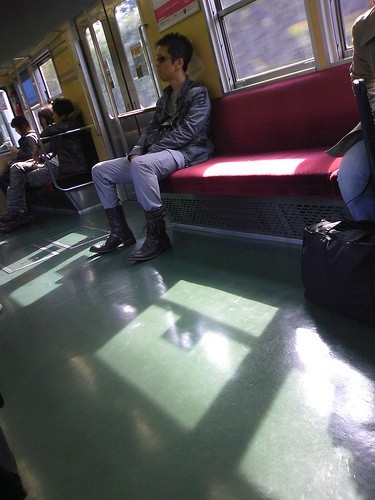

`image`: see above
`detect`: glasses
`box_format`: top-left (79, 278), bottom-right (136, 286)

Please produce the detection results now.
top-left (157, 56), bottom-right (178, 63)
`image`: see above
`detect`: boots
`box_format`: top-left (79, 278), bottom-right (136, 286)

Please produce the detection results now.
top-left (129, 207), bottom-right (171, 261)
top-left (90, 206), bottom-right (137, 252)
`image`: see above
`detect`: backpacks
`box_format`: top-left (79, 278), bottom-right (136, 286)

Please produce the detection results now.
top-left (144, 119), bottom-right (175, 150)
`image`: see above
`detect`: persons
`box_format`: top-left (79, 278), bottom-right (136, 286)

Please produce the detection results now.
top-left (0, 103), bottom-right (57, 219)
top-left (0, 98), bottom-right (96, 233)
top-left (324, 0), bottom-right (375, 221)
top-left (89, 32), bottom-right (214, 260)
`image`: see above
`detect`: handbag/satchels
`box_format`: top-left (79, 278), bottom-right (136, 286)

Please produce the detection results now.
top-left (302, 220), bottom-right (375, 324)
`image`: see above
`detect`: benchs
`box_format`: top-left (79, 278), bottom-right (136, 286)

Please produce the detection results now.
top-left (158, 62), bottom-right (375, 247)
top-left (8, 125), bottom-right (106, 215)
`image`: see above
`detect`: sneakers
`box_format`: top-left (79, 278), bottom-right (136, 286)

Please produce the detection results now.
top-left (26, 211), bottom-right (34, 223)
top-left (0, 213), bottom-right (30, 230)
